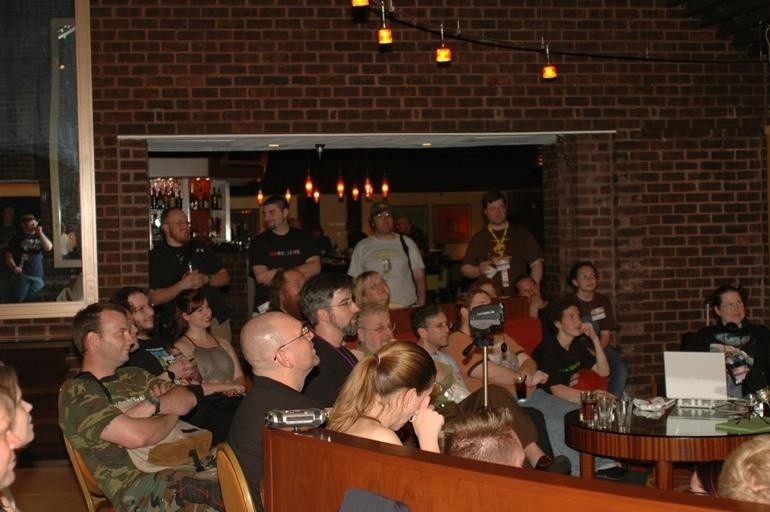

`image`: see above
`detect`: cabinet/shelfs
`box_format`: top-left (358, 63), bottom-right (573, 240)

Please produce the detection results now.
top-left (0, 333), bottom-right (82, 460)
top-left (148, 176), bottom-right (232, 251)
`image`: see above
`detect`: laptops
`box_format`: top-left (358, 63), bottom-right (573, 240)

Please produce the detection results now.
top-left (667, 406), bottom-right (736, 436)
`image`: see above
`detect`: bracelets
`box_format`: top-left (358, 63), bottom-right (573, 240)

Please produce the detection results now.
top-left (206, 273), bottom-right (212, 287)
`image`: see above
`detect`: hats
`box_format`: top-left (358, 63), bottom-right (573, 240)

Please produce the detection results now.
top-left (369, 202), bottom-right (392, 216)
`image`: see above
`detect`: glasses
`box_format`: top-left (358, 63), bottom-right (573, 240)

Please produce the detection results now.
top-left (311, 297), bottom-right (359, 314)
top-left (358, 320), bottom-right (397, 333)
top-left (421, 321), bottom-right (452, 329)
top-left (273, 321), bottom-right (314, 362)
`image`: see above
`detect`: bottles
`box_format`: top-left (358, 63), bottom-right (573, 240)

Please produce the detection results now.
top-left (189, 187), bottom-right (222, 209)
top-left (151, 186), bottom-right (182, 209)
top-left (151, 214), bottom-right (217, 239)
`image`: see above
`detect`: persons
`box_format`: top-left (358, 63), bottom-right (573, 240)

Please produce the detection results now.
top-left (394, 213), bottom-right (430, 261)
top-left (716, 432), bottom-right (770, 505)
top-left (0, 362), bottom-right (36, 512)
top-left (460, 191), bottom-right (546, 298)
top-left (59, 260), bottom-right (628, 511)
top-left (680, 283), bottom-right (769, 496)
top-left (0, 204), bottom-right (86, 301)
top-left (1, 391), bottom-right (23, 512)
top-left (146, 207), bottom-right (234, 348)
top-left (345, 201), bottom-right (427, 314)
top-left (246, 192), bottom-right (323, 320)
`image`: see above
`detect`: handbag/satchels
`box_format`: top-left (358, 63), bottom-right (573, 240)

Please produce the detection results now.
top-left (111, 393), bottom-right (212, 474)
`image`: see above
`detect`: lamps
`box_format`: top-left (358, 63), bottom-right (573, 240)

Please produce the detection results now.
top-left (350, 0), bottom-right (559, 80)
top-left (255, 158), bottom-right (390, 209)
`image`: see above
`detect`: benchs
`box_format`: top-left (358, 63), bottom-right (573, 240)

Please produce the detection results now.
top-left (262, 403), bottom-right (770, 512)
top-left (345, 317), bottom-right (608, 394)
top-left (386, 296), bottom-right (529, 333)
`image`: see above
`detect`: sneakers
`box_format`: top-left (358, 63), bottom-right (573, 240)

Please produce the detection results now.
top-left (594, 467), bottom-right (627, 481)
top-left (178, 468), bottom-right (225, 511)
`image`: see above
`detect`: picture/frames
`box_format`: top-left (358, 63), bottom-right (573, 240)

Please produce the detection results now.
top-left (432, 203), bottom-right (473, 245)
top-left (391, 204), bottom-right (429, 238)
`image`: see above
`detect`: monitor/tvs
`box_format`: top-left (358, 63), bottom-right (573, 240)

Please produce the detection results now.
top-left (663, 351), bottom-right (750, 402)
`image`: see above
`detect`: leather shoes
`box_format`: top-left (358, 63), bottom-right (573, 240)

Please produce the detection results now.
top-left (534, 453), bottom-right (571, 474)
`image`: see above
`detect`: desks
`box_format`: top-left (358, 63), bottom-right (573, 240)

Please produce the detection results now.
top-left (321, 248), bottom-right (354, 272)
top-left (565, 396), bottom-right (769, 491)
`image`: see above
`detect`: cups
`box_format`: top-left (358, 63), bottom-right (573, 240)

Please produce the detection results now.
top-left (578, 389), bottom-right (632, 426)
top-left (484, 265), bottom-right (499, 279)
top-left (185, 261), bottom-right (198, 273)
top-left (513, 376), bottom-right (528, 403)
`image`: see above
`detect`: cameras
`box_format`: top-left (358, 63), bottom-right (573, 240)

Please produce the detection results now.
top-left (468, 303), bottom-right (504, 335)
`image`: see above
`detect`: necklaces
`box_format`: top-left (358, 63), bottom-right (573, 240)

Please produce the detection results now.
top-left (485, 220), bottom-right (510, 254)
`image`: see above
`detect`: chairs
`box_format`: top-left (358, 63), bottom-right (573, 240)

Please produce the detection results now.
top-left (215, 441), bottom-right (260, 512)
top-left (62, 433), bottom-right (113, 512)
top-left (425, 263), bottom-right (471, 297)
